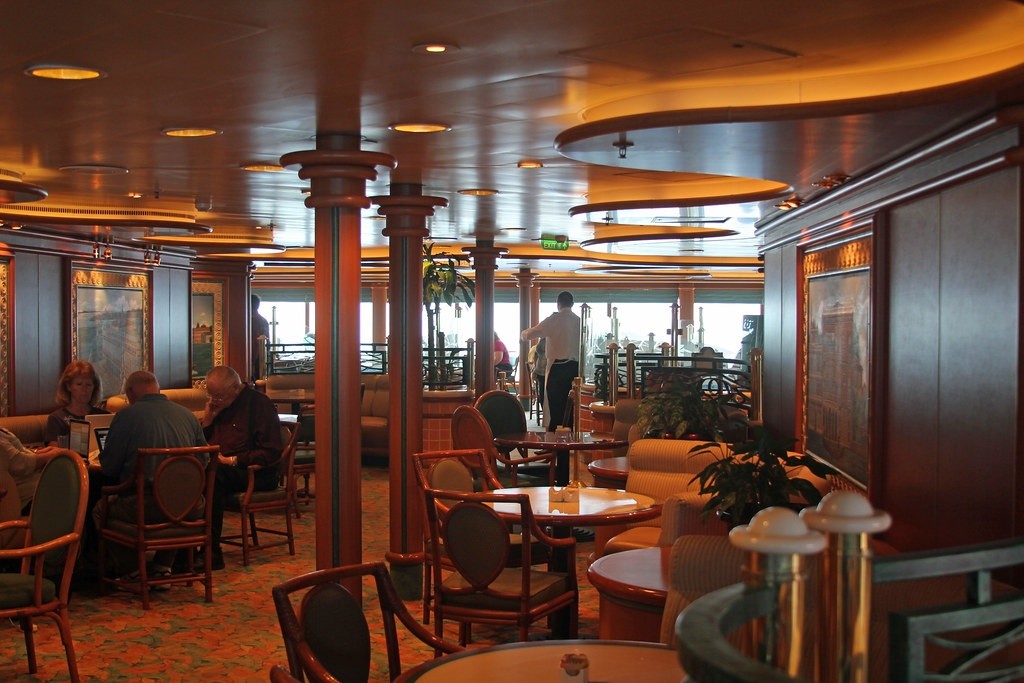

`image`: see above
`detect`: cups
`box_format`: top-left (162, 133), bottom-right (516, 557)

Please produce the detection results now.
top-left (57, 435), bottom-right (68, 448)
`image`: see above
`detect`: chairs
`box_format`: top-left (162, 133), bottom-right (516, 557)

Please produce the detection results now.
top-left (1, 390), bottom-right (319, 683)
top-left (272, 561), bottom-right (468, 683)
top-left (606, 439), bottom-right (787, 665)
top-left (413, 449), bottom-right (578, 645)
top-left (268, 373), bottom-right (392, 466)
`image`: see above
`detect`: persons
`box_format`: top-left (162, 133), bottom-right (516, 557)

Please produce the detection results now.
top-left (0, 360), bottom-right (282, 601)
top-left (250, 294), bottom-right (270, 379)
top-left (492, 291), bottom-right (581, 455)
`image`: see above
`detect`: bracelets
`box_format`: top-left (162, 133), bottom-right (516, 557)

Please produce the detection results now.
top-left (230, 456), bottom-right (235, 467)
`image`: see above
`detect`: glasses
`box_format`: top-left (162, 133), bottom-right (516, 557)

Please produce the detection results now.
top-left (206, 388), bottom-right (231, 404)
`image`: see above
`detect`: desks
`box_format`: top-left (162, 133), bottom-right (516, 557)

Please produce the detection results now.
top-left (586, 546), bottom-right (676, 642)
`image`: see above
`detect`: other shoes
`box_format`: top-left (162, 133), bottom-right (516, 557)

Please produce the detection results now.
top-left (9, 616), bottom-right (37, 633)
top-left (191, 547), bottom-right (225, 572)
top-left (129, 563), bottom-right (172, 590)
top-left (534, 449), bottom-right (551, 455)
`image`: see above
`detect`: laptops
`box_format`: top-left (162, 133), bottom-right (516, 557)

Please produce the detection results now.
top-left (94, 426), bottom-right (110, 452)
top-left (86, 414), bottom-right (116, 458)
top-left (68, 419), bottom-right (91, 465)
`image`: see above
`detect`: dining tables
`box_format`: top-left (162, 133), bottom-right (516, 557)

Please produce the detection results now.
top-left (494, 432), bottom-right (629, 486)
top-left (457, 486), bottom-right (664, 638)
top-left (392, 639), bottom-right (690, 683)
